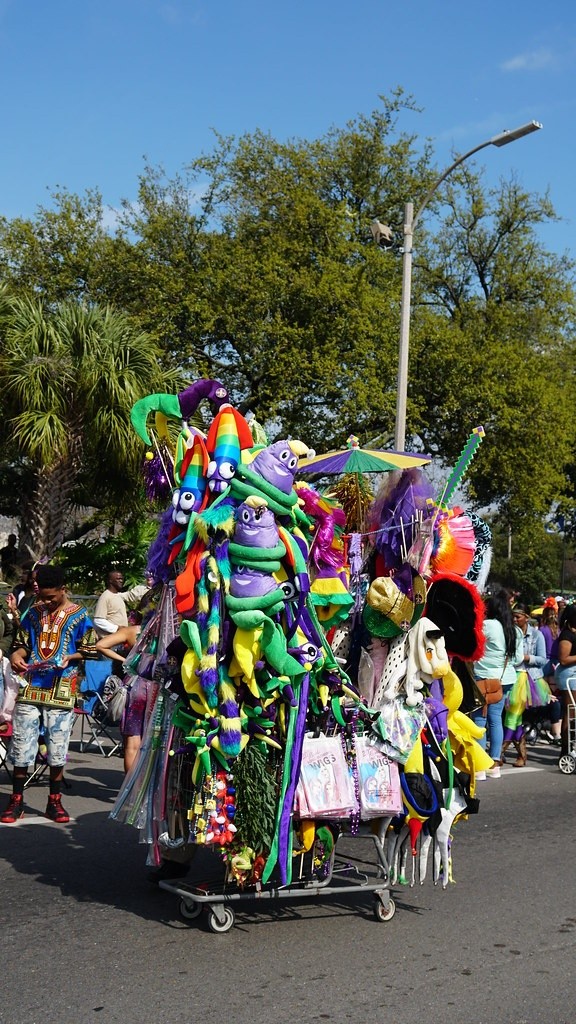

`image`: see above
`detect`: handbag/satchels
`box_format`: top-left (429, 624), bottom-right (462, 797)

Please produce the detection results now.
top-left (473, 679), bottom-right (503, 718)
top-left (93, 674), bottom-right (128, 727)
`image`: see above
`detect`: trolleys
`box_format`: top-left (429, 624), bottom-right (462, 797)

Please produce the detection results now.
top-left (558, 676), bottom-right (576, 774)
top-left (158, 809), bottom-right (397, 933)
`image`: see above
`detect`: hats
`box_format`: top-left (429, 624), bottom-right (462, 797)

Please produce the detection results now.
top-left (362, 562), bottom-right (426, 638)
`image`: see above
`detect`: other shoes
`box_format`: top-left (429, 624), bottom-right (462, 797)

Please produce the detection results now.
top-left (474, 771), bottom-right (487, 780)
top-left (554, 738), bottom-right (565, 747)
top-left (487, 766), bottom-right (501, 779)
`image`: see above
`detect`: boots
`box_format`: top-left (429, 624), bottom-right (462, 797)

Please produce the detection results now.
top-left (491, 740), bottom-right (510, 768)
top-left (511, 733), bottom-right (527, 767)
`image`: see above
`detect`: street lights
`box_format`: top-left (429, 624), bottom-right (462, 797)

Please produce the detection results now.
top-left (390, 118), bottom-right (545, 491)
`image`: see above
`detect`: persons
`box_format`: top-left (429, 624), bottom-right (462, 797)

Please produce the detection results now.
top-left (0, 565), bottom-right (100, 822)
top-left (471, 589), bottom-right (576, 780)
top-left (0, 534), bottom-right (159, 773)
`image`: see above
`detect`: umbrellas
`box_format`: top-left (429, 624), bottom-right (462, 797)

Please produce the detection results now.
top-left (297, 435), bottom-right (433, 528)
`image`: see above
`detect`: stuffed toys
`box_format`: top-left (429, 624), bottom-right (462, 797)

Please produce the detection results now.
top-left (384, 618), bottom-right (448, 707)
top-left (129, 377), bottom-right (326, 785)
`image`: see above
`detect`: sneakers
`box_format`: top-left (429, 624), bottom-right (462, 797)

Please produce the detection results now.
top-left (46, 792), bottom-right (70, 823)
top-left (1, 794), bottom-right (25, 823)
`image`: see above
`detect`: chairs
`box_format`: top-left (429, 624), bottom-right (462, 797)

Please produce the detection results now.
top-left (0, 738), bottom-right (73, 790)
top-left (76, 659), bottom-right (126, 758)
